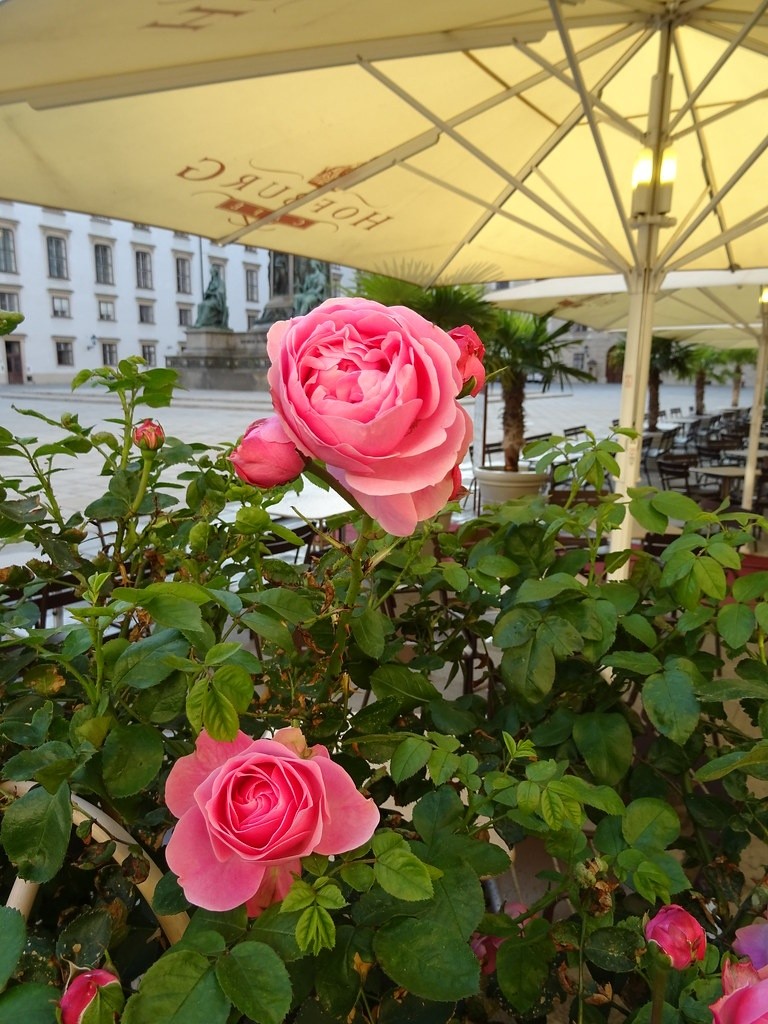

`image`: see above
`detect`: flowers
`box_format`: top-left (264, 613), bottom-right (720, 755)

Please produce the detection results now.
top-left (0, 296), bottom-right (768, 1024)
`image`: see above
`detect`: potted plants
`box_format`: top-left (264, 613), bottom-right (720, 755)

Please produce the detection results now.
top-left (474, 307), bottom-right (596, 514)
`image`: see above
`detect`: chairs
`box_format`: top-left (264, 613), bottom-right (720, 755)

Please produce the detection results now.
top-left (464, 406), bottom-right (750, 512)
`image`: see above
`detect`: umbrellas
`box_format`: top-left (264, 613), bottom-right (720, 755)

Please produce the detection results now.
top-left (0, 0), bottom-right (768, 687)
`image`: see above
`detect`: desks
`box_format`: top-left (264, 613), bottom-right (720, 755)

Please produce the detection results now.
top-left (519, 407), bottom-right (768, 500)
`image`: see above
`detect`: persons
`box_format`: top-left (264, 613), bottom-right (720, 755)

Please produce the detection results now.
top-left (192, 266), bottom-right (229, 328)
top-left (293, 259), bottom-right (327, 317)
